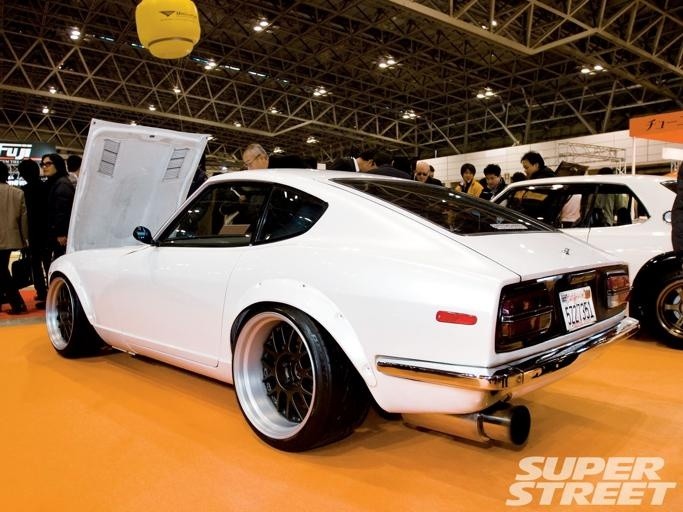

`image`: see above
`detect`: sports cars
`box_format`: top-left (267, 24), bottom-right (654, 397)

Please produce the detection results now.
top-left (42, 115), bottom-right (641, 455)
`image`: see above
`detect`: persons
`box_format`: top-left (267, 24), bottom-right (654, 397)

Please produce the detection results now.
top-left (170, 163), bottom-right (212, 238)
top-left (220, 140), bottom-right (627, 240)
top-left (0, 153), bottom-right (82, 316)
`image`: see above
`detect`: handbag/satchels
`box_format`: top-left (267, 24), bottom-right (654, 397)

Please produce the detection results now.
top-left (11, 249), bottom-right (34, 290)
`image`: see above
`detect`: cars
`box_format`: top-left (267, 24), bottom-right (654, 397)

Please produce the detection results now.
top-left (490, 173), bottom-right (680, 348)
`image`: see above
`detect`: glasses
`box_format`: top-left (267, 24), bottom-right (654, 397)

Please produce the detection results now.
top-left (243, 151), bottom-right (262, 169)
top-left (416, 171), bottom-right (429, 177)
top-left (39, 161), bottom-right (54, 168)
